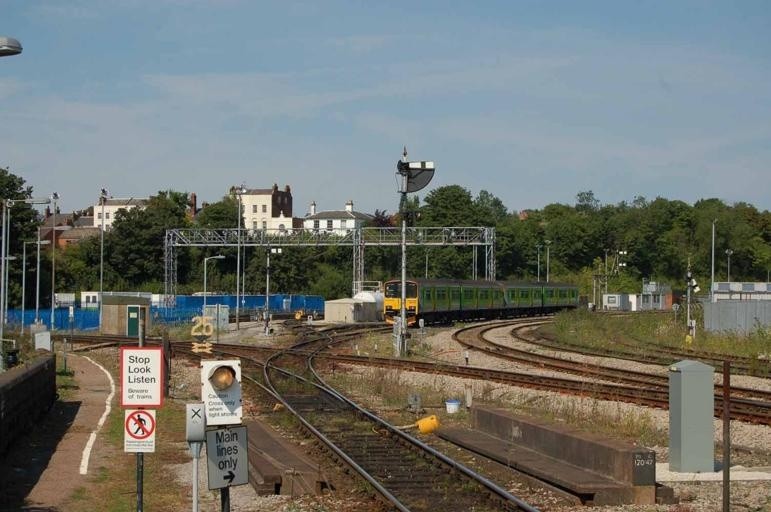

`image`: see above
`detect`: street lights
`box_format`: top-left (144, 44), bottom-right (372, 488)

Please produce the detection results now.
top-left (35, 225), bottom-right (73, 320)
top-left (529, 219), bottom-right (733, 301)
top-left (262, 240), bottom-right (284, 334)
top-left (203, 255), bottom-right (226, 316)
top-left (0, 255), bottom-right (18, 261)
top-left (99, 187), bottom-right (107, 332)
top-left (50, 191), bottom-right (60, 329)
top-left (21, 240), bottom-right (52, 334)
top-left (0, 198), bottom-right (52, 351)
top-left (235, 186), bottom-right (248, 331)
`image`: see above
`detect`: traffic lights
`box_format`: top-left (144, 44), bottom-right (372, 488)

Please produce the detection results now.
top-left (201, 359), bottom-right (243, 426)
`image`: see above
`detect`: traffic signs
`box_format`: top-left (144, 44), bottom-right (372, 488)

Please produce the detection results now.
top-left (202, 426), bottom-right (251, 490)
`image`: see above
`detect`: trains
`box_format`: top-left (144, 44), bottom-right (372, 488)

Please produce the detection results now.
top-left (383, 278), bottom-right (581, 326)
